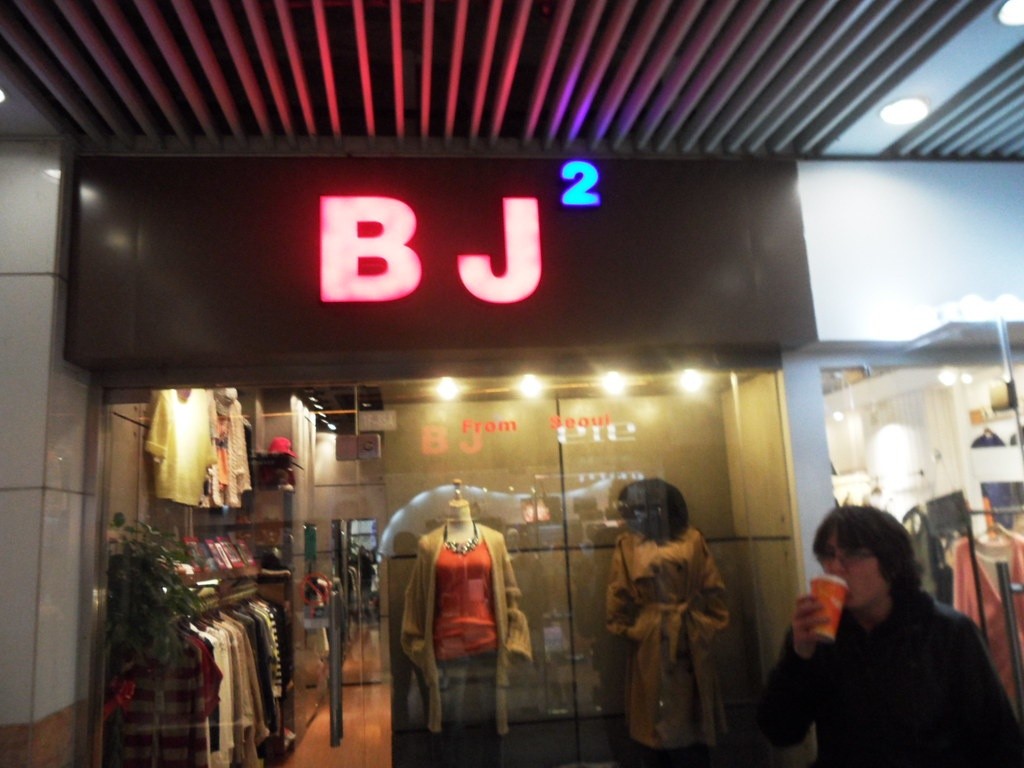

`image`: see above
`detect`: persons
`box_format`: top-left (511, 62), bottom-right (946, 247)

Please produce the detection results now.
top-left (758, 504), bottom-right (1024, 768)
top-left (401, 500), bottom-right (533, 768)
top-left (606, 478), bottom-right (744, 768)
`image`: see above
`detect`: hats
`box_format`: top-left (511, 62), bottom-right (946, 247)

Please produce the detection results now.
top-left (215, 387), bottom-right (238, 415)
top-left (617, 479), bottom-right (690, 537)
top-left (268, 437), bottom-right (297, 458)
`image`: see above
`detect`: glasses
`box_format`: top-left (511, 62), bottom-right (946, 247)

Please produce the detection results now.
top-left (819, 547), bottom-right (876, 562)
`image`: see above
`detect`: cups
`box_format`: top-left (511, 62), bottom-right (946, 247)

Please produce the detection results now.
top-left (808, 574), bottom-right (848, 640)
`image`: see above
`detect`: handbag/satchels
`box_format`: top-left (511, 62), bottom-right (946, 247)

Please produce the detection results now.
top-left (970, 377), bottom-right (1024, 448)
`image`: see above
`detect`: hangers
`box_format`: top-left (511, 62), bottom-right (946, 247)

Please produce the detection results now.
top-left (973, 508), bottom-right (1015, 545)
top-left (168, 583), bottom-right (261, 640)
